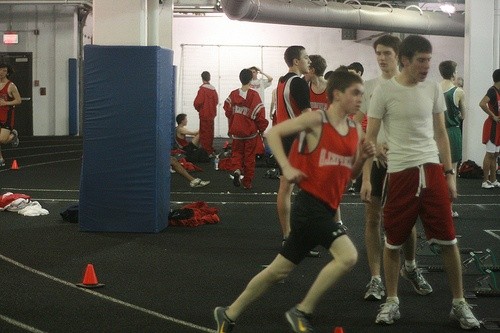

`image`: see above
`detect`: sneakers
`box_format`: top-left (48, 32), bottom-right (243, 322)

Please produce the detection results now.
top-left (364, 277), bottom-right (385, 300)
top-left (214, 306), bottom-right (235, 333)
top-left (481, 179), bottom-right (500, 188)
top-left (450, 301), bottom-right (480, 329)
top-left (0, 161), bottom-right (7, 170)
top-left (285, 306), bottom-right (316, 333)
top-left (10, 129), bottom-right (19, 147)
top-left (400, 264), bottom-right (433, 295)
top-left (375, 298), bottom-right (400, 324)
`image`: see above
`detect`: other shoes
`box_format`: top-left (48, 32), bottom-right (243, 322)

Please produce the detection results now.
top-left (347, 187), bottom-right (356, 196)
top-left (240, 177), bottom-right (254, 189)
top-left (233, 169), bottom-right (241, 187)
top-left (280, 238), bottom-right (321, 258)
top-left (190, 177), bottom-right (210, 188)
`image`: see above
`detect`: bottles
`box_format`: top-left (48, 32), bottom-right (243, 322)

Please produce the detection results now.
top-left (214, 155), bottom-right (220, 170)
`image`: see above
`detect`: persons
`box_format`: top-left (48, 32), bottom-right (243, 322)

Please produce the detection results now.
top-left (223, 68), bottom-right (269, 190)
top-left (214, 70), bottom-right (376, 332)
top-left (275, 45), bottom-right (349, 257)
top-left (174, 113), bottom-right (222, 159)
top-left (359, 35), bottom-right (481, 331)
top-left (0, 61), bottom-right (22, 170)
top-left (193, 71), bottom-right (218, 158)
top-left (248, 66), bottom-right (274, 161)
top-left (479, 69), bottom-right (500, 189)
top-left (269, 45), bottom-right (367, 192)
top-left (352, 34), bottom-right (434, 300)
top-left (439, 60), bottom-right (465, 219)
top-left (171, 122), bottom-right (210, 189)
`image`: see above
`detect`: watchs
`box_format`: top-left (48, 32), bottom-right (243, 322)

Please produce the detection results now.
top-left (445, 168), bottom-right (455, 175)
